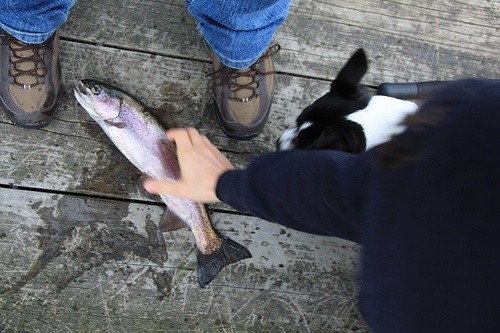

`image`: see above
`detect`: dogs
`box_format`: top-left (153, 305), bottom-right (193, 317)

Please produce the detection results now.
top-left (275, 46), bottom-right (420, 154)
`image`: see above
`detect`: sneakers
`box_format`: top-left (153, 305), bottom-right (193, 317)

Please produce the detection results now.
top-left (206, 48), bottom-right (278, 145)
top-left (0, 31), bottom-right (62, 128)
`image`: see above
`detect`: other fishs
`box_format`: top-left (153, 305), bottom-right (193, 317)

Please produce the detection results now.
top-left (73, 78), bottom-right (253, 289)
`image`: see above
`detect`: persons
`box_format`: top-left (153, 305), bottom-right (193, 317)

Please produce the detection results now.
top-left (0, 0), bottom-right (292, 143)
top-left (143, 71), bottom-right (500, 333)
